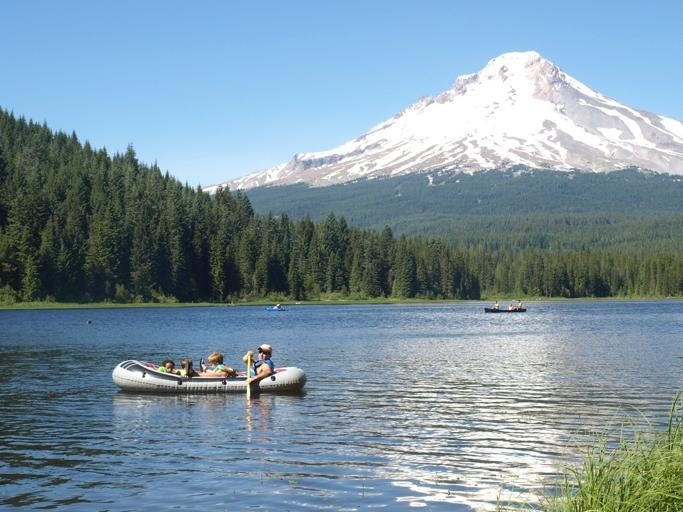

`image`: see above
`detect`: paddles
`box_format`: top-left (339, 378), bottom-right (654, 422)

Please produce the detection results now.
top-left (246, 355), bottom-right (251, 401)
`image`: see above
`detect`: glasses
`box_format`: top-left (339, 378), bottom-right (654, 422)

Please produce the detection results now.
top-left (258, 350), bottom-right (267, 354)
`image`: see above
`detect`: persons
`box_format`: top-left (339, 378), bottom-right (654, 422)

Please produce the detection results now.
top-left (242, 342), bottom-right (274, 383)
top-left (157, 360), bottom-right (174, 373)
top-left (273, 303), bottom-right (280, 309)
top-left (490, 300), bottom-right (498, 309)
top-left (507, 304), bottom-right (513, 311)
top-left (515, 300), bottom-right (522, 308)
top-left (199, 352), bottom-right (235, 379)
top-left (174, 357), bottom-right (197, 376)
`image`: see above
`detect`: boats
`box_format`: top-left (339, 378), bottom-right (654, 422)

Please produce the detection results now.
top-left (264, 306), bottom-right (289, 311)
top-left (483, 306), bottom-right (527, 313)
top-left (110, 357), bottom-right (305, 395)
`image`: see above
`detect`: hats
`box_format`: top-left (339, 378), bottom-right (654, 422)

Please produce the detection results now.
top-left (257, 344), bottom-right (272, 355)
